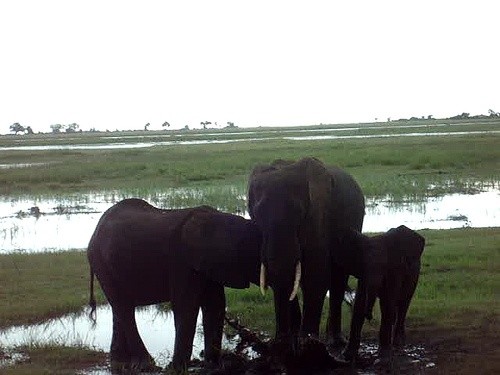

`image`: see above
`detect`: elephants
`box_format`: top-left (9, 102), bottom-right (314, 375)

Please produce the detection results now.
top-left (334, 223), bottom-right (426, 369)
top-left (247, 154), bottom-right (366, 348)
top-left (86, 197), bottom-right (302, 374)
